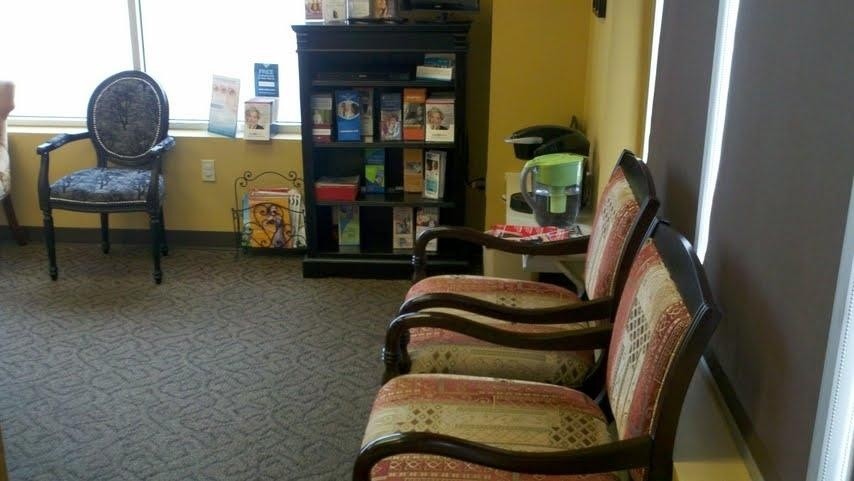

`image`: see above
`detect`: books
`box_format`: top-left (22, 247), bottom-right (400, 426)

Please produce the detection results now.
top-left (302, 0), bottom-right (458, 251)
top-left (244, 100), bottom-right (273, 141)
top-left (240, 187), bottom-right (301, 248)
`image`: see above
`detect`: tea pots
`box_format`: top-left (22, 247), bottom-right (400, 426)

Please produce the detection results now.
top-left (519, 153), bottom-right (588, 230)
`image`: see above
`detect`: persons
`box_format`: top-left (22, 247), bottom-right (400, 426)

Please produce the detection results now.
top-left (249, 110), bottom-right (264, 129)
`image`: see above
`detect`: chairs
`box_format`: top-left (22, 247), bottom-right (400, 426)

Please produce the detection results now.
top-left (389, 147), bottom-right (662, 388)
top-left (0, 69), bottom-right (175, 285)
top-left (351, 218), bottom-right (721, 481)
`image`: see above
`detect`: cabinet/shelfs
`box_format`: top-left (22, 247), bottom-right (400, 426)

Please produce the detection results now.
top-left (291, 0), bottom-right (493, 281)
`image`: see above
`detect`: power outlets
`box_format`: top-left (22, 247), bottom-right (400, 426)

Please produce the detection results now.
top-left (202, 159), bottom-right (215, 181)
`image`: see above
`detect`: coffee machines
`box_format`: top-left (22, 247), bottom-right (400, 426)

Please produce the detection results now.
top-left (505, 116), bottom-right (590, 213)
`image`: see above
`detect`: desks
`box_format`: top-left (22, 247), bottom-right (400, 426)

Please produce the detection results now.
top-left (506, 172), bottom-right (594, 296)
